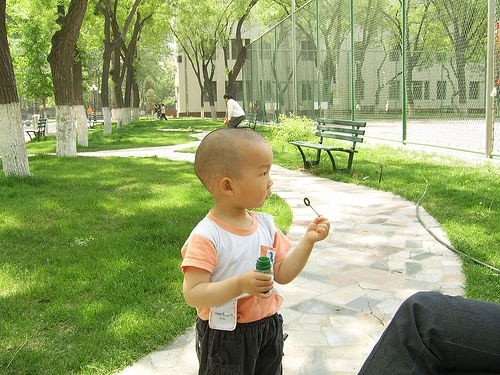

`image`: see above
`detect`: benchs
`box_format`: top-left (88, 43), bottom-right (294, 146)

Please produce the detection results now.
top-left (88, 115), bottom-right (106, 127)
top-left (26, 119), bottom-right (46, 142)
top-left (289, 117), bottom-right (366, 174)
top-left (238, 111), bottom-right (256, 131)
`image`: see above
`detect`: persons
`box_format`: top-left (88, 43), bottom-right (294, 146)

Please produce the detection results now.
top-left (180, 127), bottom-right (330, 375)
top-left (153, 104), bottom-right (168, 121)
top-left (358, 290), bottom-right (500, 375)
top-left (223, 95), bottom-right (246, 128)
top-left (87, 105), bottom-right (93, 127)
